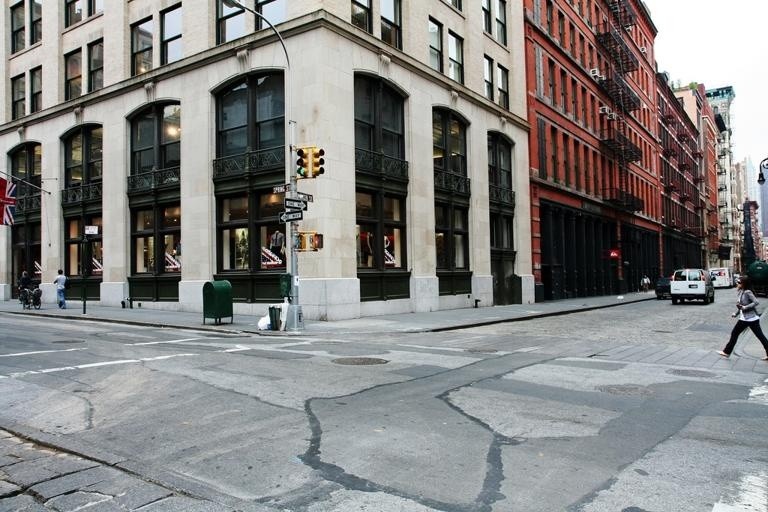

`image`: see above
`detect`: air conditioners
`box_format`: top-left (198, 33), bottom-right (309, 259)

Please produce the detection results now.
top-left (641, 47), bottom-right (646, 54)
top-left (599, 106), bottom-right (617, 121)
top-left (625, 23), bottom-right (632, 32)
top-left (591, 69), bottom-right (606, 83)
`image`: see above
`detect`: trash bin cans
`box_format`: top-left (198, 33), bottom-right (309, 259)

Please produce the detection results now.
top-left (203, 279), bottom-right (233, 325)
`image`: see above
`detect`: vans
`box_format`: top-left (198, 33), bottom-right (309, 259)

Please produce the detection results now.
top-left (655, 268), bottom-right (742, 304)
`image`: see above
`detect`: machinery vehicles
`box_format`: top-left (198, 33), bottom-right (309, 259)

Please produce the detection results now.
top-left (743, 260), bottom-right (768, 297)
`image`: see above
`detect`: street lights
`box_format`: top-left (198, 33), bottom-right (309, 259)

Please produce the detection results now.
top-left (758, 157), bottom-right (768, 185)
top-left (221, 0), bottom-right (305, 331)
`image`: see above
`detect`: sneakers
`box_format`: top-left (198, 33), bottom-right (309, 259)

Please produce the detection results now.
top-left (715, 349), bottom-right (729, 358)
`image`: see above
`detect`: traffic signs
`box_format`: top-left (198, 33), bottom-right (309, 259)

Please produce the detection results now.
top-left (273, 183), bottom-right (312, 224)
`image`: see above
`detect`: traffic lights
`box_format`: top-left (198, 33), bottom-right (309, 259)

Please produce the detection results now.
top-left (294, 230), bottom-right (324, 253)
top-left (295, 146), bottom-right (326, 179)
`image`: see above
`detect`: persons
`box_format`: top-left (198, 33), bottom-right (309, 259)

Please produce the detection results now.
top-left (16, 270), bottom-right (31, 305)
top-left (367, 231), bottom-right (374, 268)
top-left (269, 230), bottom-right (285, 260)
top-left (715, 276), bottom-right (768, 361)
top-left (53, 269), bottom-right (69, 309)
top-left (640, 273), bottom-right (651, 292)
top-left (383, 235), bottom-right (391, 249)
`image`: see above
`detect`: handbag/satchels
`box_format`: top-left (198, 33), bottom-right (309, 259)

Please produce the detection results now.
top-left (754, 303), bottom-right (764, 317)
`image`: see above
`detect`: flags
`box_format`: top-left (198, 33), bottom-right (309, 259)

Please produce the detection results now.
top-left (0, 177), bottom-right (17, 227)
